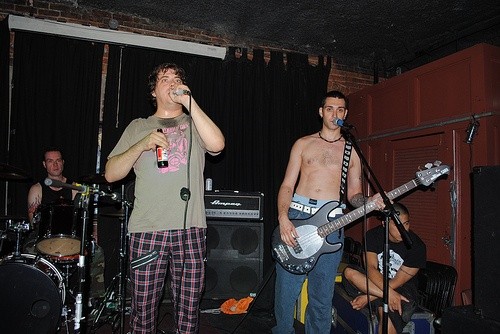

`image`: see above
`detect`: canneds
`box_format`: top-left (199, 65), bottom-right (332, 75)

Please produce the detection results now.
top-left (206, 178), bottom-right (212, 191)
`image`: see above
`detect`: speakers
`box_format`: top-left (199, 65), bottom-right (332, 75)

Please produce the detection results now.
top-left (467, 166), bottom-right (500, 322)
top-left (441, 305), bottom-right (500, 334)
top-left (200, 222), bottom-right (276, 308)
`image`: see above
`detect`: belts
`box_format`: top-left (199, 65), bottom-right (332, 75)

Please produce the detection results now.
top-left (289, 202), bottom-right (342, 218)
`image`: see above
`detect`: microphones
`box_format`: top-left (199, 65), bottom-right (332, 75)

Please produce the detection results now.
top-left (465, 122), bottom-right (477, 143)
top-left (333, 118), bottom-right (355, 128)
top-left (172, 89), bottom-right (189, 95)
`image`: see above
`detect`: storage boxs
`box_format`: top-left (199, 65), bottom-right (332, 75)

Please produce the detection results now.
top-left (330, 280), bottom-right (435, 334)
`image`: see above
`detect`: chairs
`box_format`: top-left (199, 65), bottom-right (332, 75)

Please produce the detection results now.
top-left (343, 237), bottom-right (458, 329)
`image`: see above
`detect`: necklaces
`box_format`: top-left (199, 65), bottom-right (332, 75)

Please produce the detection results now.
top-left (319, 132), bottom-right (343, 143)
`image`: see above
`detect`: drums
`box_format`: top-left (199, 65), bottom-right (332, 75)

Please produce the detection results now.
top-left (34, 204), bottom-right (90, 257)
top-left (0, 253), bottom-right (67, 334)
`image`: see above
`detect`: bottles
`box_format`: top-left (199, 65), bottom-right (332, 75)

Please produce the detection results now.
top-left (156, 128), bottom-right (168, 168)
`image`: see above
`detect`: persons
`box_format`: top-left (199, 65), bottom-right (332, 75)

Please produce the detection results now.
top-left (342, 203), bottom-right (427, 334)
top-left (27, 147), bottom-right (83, 241)
top-left (105, 63), bottom-right (225, 334)
top-left (272, 90), bottom-right (394, 334)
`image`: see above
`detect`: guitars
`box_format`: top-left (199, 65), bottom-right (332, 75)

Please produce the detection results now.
top-left (269, 161), bottom-right (450, 276)
